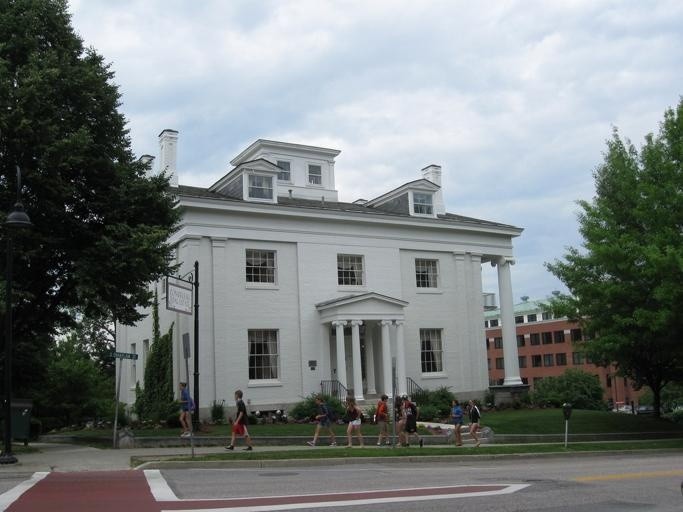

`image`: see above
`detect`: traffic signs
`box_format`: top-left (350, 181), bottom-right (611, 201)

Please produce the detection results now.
top-left (105, 352), bottom-right (138, 361)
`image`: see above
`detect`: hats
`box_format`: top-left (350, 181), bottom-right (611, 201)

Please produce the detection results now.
top-left (402, 395), bottom-right (409, 401)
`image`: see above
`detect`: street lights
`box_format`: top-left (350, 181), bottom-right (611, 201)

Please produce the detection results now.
top-left (0, 163), bottom-right (34, 464)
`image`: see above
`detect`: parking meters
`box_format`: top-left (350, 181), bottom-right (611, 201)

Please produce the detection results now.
top-left (561, 400), bottom-right (574, 449)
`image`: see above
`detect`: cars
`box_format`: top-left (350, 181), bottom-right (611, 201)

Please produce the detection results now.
top-left (611, 403), bottom-right (673, 414)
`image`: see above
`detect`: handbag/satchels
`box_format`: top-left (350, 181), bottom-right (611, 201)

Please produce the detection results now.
top-left (189, 398), bottom-right (196, 410)
top-left (370, 412), bottom-right (377, 425)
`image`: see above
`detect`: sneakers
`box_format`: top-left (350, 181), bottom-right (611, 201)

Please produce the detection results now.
top-left (419, 438), bottom-right (423, 448)
top-left (224, 444), bottom-right (234, 450)
top-left (457, 440), bottom-right (481, 447)
top-left (376, 441), bottom-right (409, 448)
top-left (307, 441), bottom-right (315, 447)
top-left (242, 445), bottom-right (253, 450)
top-left (180, 430), bottom-right (194, 438)
top-left (329, 442), bottom-right (337, 446)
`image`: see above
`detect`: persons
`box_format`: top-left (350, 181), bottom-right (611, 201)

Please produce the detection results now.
top-left (451, 400), bottom-right (464, 446)
top-left (467, 399), bottom-right (482, 448)
top-left (344, 396), bottom-right (364, 449)
top-left (225, 390), bottom-right (253, 451)
top-left (178, 381), bottom-right (195, 438)
top-left (394, 394), bottom-right (423, 447)
top-left (376, 395), bottom-right (392, 446)
top-left (306, 396), bottom-right (337, 447)
